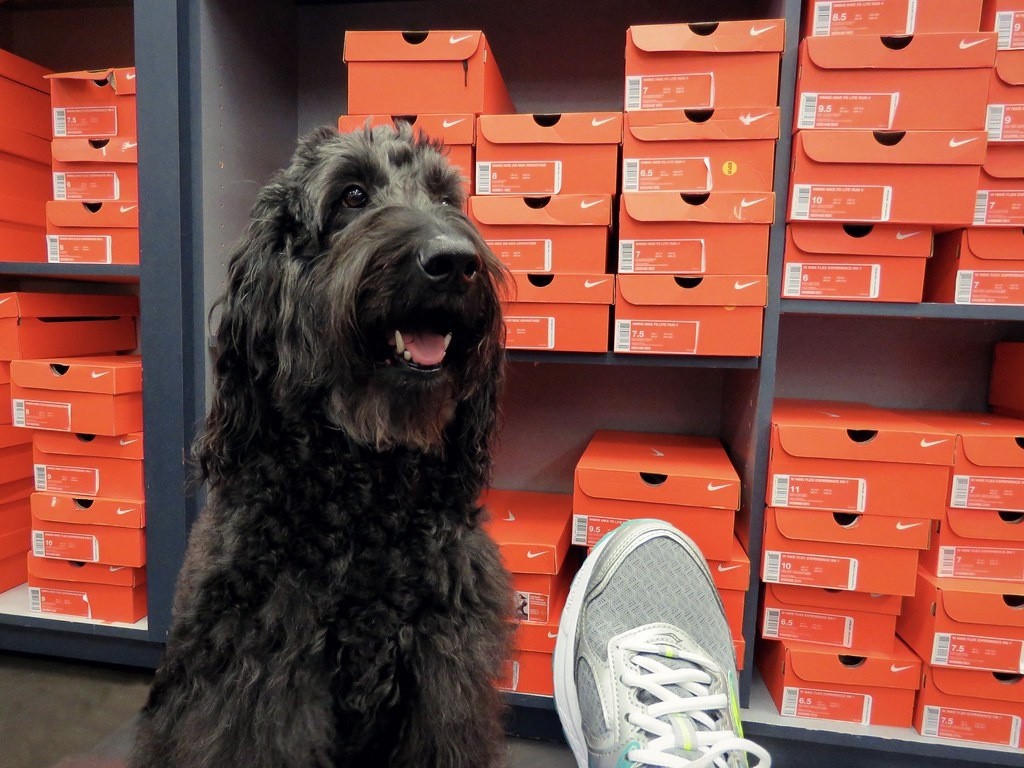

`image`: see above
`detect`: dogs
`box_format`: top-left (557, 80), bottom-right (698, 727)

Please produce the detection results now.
top-left (121, 115), bottom-right (521, 767)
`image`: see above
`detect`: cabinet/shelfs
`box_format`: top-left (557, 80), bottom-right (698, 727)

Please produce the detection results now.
top-left (1, 0), bottom-right (1024, 768)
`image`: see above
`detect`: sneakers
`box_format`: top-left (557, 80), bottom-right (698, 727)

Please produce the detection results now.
top-left (552, 518), bottom-right (772, 768)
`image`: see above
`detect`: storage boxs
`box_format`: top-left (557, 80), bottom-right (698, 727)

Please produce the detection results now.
top-left (0, 291), bottom-right (146, 624)
top-left (3, 48), bottom-right (140, 265)
top-left (614, 17), bottom-right (785, 357)
top-left (755, 344), bottom-right (1024, 752)
top-left (476, 429), bottom-right (751, 697)
top-left (780, 0), bottom-right (1024, 308)
top-left (339, 30), bottom-right (622, 353)
